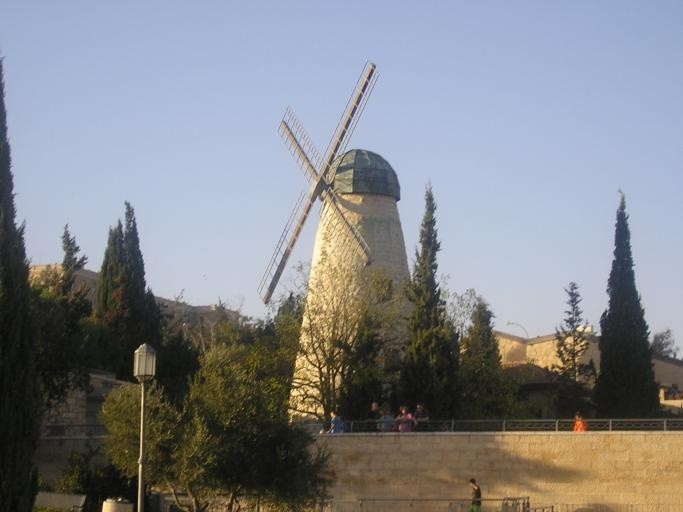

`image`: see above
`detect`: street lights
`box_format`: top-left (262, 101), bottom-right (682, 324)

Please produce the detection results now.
top-left (131, 342), bottom-right (157, 512)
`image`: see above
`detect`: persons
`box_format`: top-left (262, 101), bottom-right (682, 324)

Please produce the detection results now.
top-left (366, 401), bottom-right (384, 431)
top-left (395, 402), bottom-right (418, 431)
top-left (318, 428), bottom-right (333, 434)
top-left (413, 402), bottom-right (430, 430)
top-left (572, 410), bottom-right (585, 432)
top-left (376, 401), bottom-right (395, 431)
top-left (326, 410), bottom-right (345, 435)
top-left (469, 478), bottom-right (481, 511)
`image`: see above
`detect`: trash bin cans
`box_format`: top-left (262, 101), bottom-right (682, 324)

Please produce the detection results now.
top-left (101, 497), bottom-right (134, 512)
